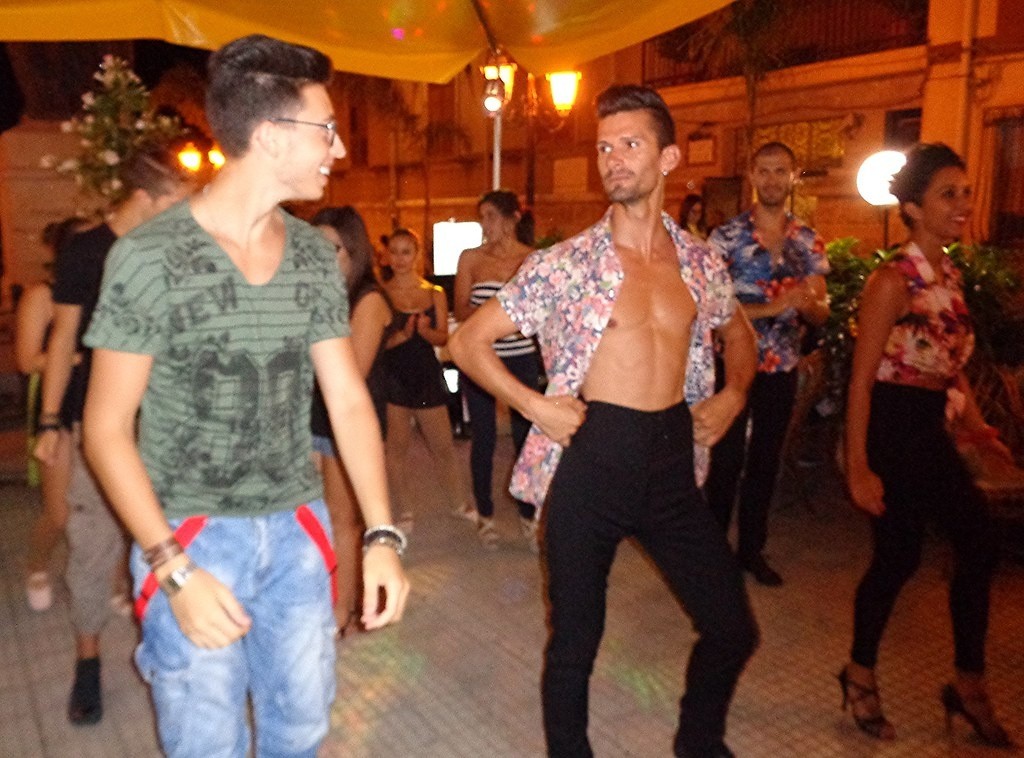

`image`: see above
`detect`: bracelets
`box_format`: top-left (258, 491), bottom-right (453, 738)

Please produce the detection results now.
top-left (362, 530), bottom-right (402, 556)
top-left (363, 530), bottom-right (406, 550)
top-left (362, 523), bottom-right (409, 549)
top-left (139, 538), bottom-right (181, 571)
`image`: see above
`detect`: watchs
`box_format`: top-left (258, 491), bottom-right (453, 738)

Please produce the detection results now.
top-left (161, 560), bottom-right (198, 596)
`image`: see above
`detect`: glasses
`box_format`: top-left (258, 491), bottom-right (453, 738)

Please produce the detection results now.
top-left (268, 115), bottom-right (338, 147)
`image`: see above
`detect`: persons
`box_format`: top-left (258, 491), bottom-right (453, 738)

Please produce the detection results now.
top-left (841, 142), bottom-right (1015, 749)
top-left (19, 148), bottom-right (708, 725)
top-left (703, 142), bottom-right (833, 585)
top-left (82, 34), bottom-right (410, 758)
top-left (445, 85), bottom-right (761, 758)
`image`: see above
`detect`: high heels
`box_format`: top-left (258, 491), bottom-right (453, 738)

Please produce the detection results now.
top-left (939, 683), bottom-right (1015, 748)
top-left (838, 666), bottom-right (899, 741)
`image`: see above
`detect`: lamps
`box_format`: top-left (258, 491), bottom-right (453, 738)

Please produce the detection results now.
top-left (856, 150), bottom-right (908, 251)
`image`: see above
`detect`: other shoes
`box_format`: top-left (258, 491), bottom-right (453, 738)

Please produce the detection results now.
top-left (335, 609), bottom-right (363, 639)
top-left (519, 515), bottom-right (540, 553)
top-left (474, 517), bottom-right (500, 552)
top-left (22, 571), bottom-right (52, 612)
top-left (397, 512), bottom-right (414, 536)
top-left (110, 594), bottom-right (132, 617)
top-left (68, 656), bottom-right (103, 724)
top-left (450, 502), bottom-right (479, 521)
top-left (736, 551), bottom-right (781, 586)
